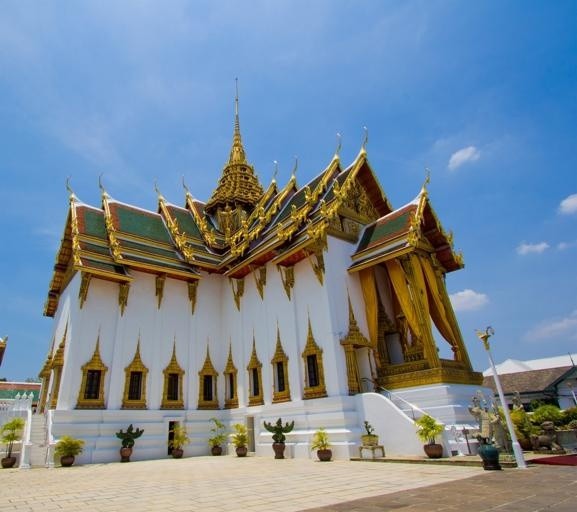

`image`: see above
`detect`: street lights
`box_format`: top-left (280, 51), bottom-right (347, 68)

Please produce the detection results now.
top-left (475, 325), bottom-right (528, 469)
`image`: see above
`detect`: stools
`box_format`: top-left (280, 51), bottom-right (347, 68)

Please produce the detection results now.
top-left (359, 446), bottom-right (384, 459)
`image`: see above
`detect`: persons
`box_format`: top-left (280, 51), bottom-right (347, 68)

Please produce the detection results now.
top-left (512, 395), bottom-right (526, 413)
top-left (467, 402), bottom-right (497, 445)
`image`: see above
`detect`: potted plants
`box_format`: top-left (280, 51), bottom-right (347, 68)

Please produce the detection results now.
top-left (309, 426), bottom-right (335, 462)
top-left (0, 419), bottom-right (23, 469)
top-left (54, 434), bottom-right (85, 467)
top-left (415, 415), bottom-right (445, 457)
top-left (261, 417), bottom-right (296, 461)
top-left (116, 422), bottom-right (144, 463)
top-left (359, 422), bottom-right (380, 448)
top-left (210, 418), bottom-right (248, 457)
top-left (167, 419), bottom-right (187, 459)
